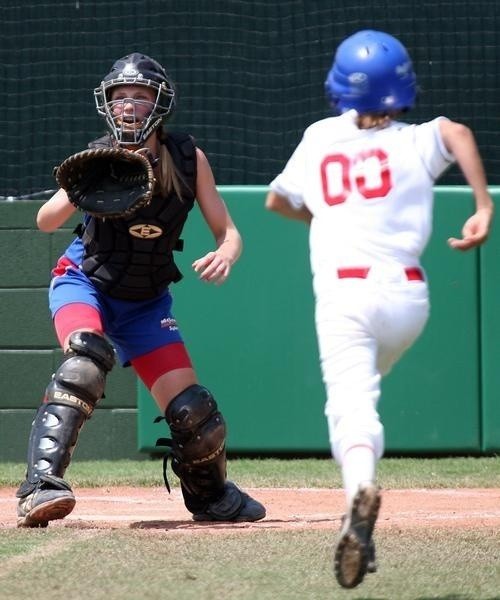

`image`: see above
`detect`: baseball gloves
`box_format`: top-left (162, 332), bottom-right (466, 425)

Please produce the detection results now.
top-left (56, 146), bottom-right (155, 221)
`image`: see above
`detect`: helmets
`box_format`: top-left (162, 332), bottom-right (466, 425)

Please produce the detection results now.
top-left (325, 28), bottom-right (418, 114)
top-left (92, 52), bottom-right (176, 147)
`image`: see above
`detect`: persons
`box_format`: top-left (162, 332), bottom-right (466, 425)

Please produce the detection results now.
top-left (16, 50), bottom-right (267, 527)
top-left (264, 27), bottom-right (494, 591)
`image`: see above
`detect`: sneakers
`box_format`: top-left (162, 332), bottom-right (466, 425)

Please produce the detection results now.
top-left (191, 483), bottom-right (267, 525)
top-left (16, 486), bottom-right (77, 529)
top-left (332, 483), bottom-right (383, 590)
